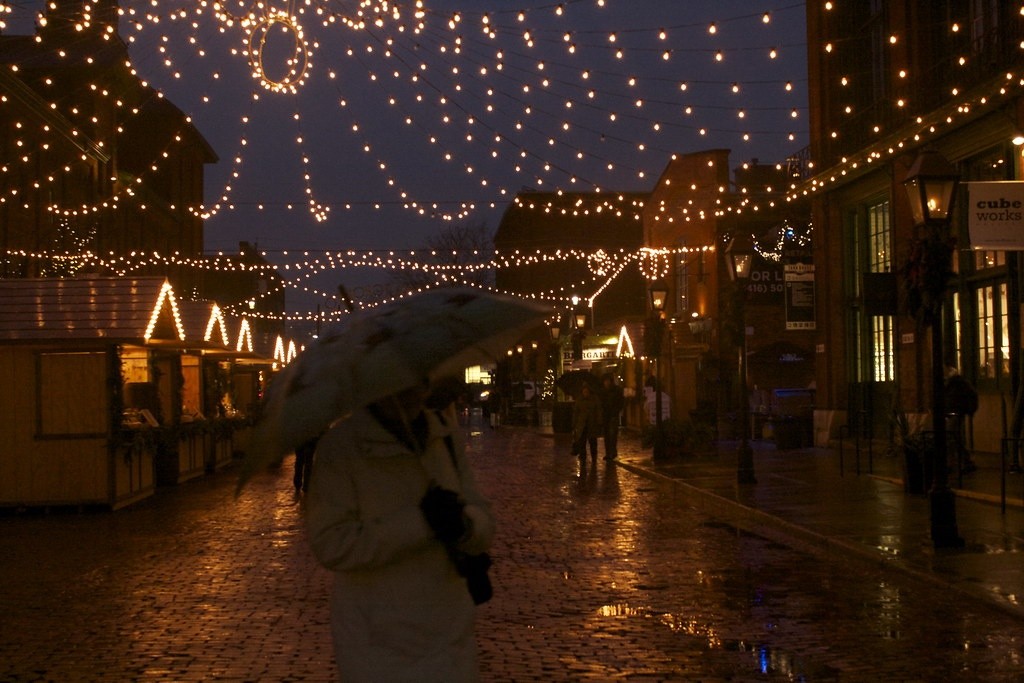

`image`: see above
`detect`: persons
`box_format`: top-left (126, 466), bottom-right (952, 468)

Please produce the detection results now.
top-left (573, 373), bottom-right (623, 479)
top-left (488, 387), bottom-right (501, 430)
top-left (943, 362), bottom-right (979, 472)
top-left (306, 378), bottom-right (495, 683)
top-left (294, 435), bottom-right (318, 495)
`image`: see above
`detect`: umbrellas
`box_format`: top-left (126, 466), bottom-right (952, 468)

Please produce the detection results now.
top-left (556, 370), bottom-right (602, 402)
top-left (235, 284), bottom-right (552, 487)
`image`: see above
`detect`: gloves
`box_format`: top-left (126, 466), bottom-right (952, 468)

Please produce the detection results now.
top-left (420, 487), bottom-right (466, 542)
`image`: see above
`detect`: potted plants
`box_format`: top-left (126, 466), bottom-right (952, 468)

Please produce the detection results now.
top-left (888, 403), bottom-right (939, 497)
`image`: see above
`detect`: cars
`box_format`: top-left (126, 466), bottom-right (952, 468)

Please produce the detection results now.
top-left (512, 380), bottom-right (543, 404)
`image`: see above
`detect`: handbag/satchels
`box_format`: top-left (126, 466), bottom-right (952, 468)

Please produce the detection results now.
top-left (572, 441), bottom-right (580, 456)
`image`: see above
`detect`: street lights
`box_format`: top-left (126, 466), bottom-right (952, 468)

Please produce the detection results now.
top-left (575, 303), bottom-right (586, 361)
top-left (649, 272), bottom-right (668, 457)
top-left (547, 317), bottom-right (563, 405)
top-left (903, 134), bottom-right (954, 551)
top-left (724, 228), bottom-right (762, 485)
